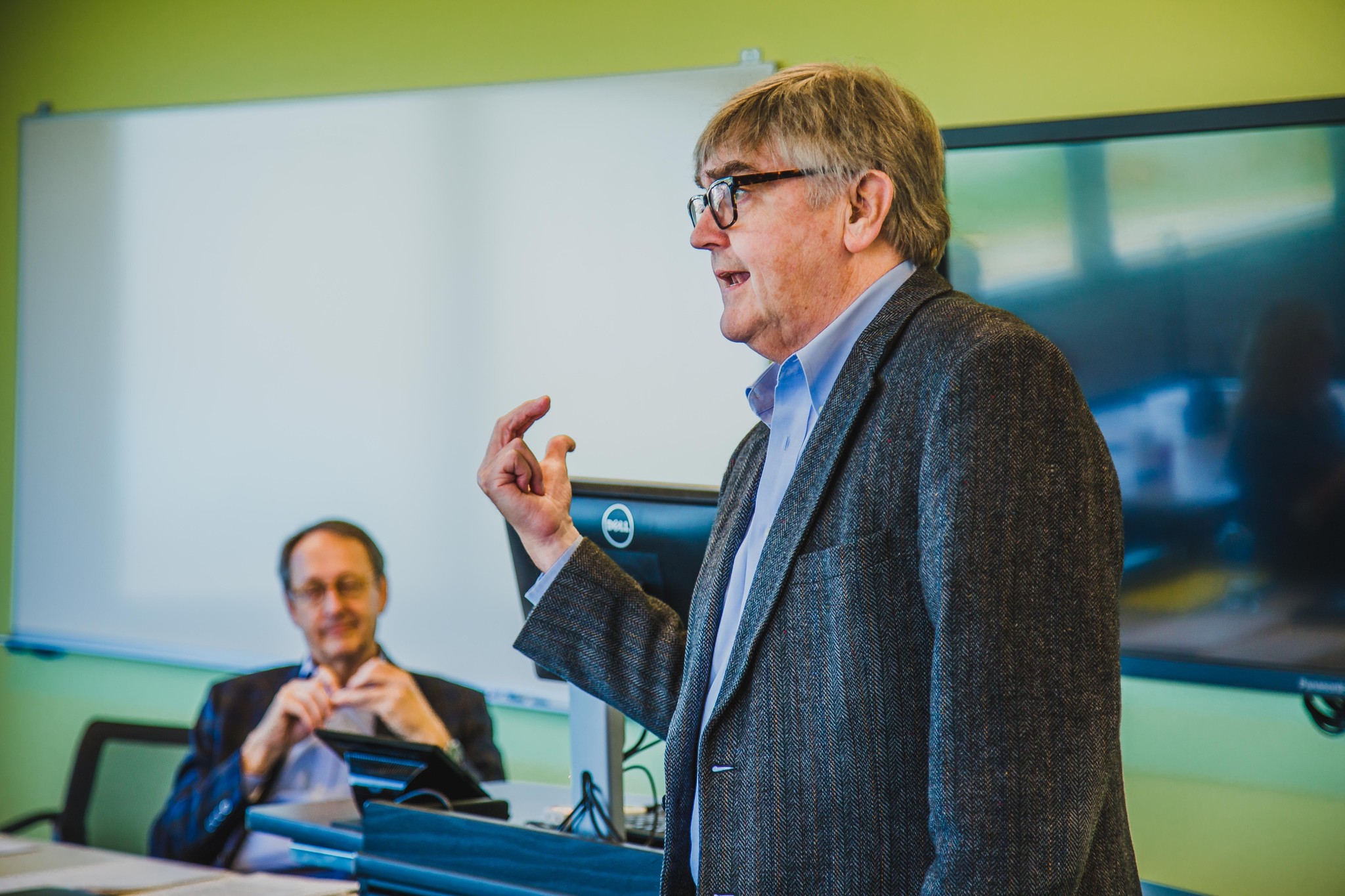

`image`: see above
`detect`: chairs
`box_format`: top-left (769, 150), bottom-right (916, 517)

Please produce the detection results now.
top-left (2, 720), bottom-right (197, 847)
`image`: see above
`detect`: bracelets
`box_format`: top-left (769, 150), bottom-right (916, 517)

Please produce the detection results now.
top-left (447, 743), bottom-right (461, 764)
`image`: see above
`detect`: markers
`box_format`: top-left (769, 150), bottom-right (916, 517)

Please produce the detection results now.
top-left (507, 692), bottom-right (548, 706)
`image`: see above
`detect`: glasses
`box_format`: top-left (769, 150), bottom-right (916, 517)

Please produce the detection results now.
top-left (687, 166), bottom-right (857, 231)
top-left (287, 572), bottom-right (378, 607)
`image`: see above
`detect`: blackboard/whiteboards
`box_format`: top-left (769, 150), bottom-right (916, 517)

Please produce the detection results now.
top-left (3, 56), bottom-right (786, 716)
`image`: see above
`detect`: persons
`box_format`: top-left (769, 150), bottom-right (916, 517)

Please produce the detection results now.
top-left (478, 61), bottom-right (1142, 896)
top-left (148, 521), bottom-right (507, 883)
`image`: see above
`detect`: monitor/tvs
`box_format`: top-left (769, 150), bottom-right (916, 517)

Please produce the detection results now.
top-left (936, 96), bottom-right (1345, 697)
top-left (503, 484), bottom-right (720, 683)
